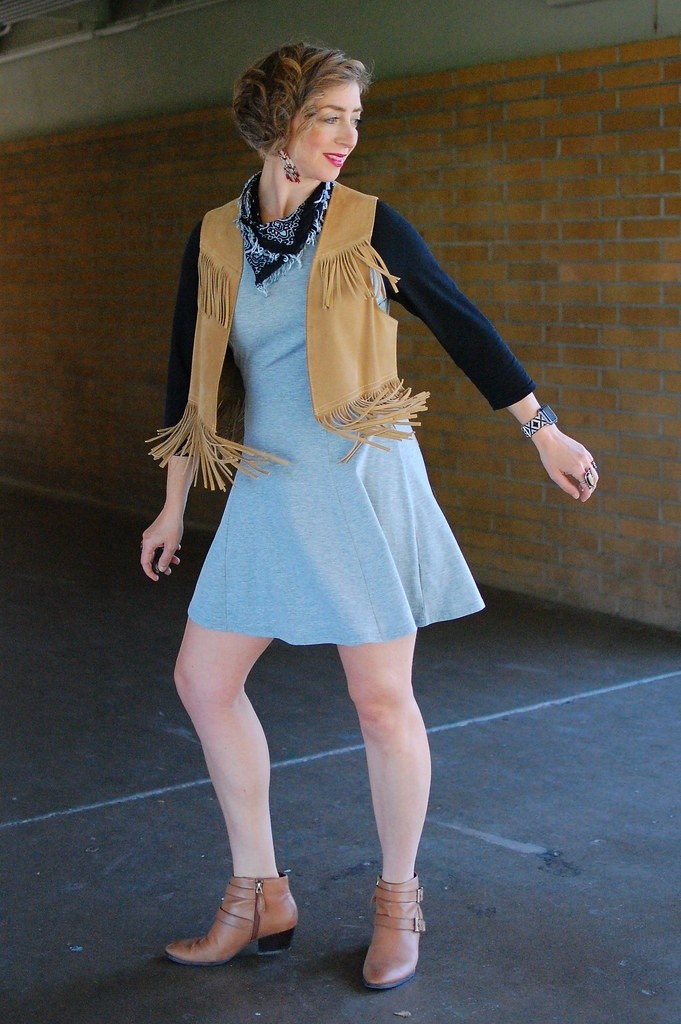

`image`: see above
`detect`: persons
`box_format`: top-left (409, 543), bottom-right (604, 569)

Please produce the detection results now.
top-left (140, 39), bottom-right (599, 988)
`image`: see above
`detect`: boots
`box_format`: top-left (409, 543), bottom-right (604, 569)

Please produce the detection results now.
top-left (164, 870), bottom-right (298, 968)
top-left (361, 875), bottom-right (428, 989)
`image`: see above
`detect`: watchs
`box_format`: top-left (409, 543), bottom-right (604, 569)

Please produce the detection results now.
top-left (520, 404), bottom-right (558, 440)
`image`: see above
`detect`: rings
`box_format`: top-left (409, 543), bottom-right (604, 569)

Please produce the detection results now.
top-left (591, 461), bottom-right (598, 469)
top-left (580, 472), bottom-right (595, 488)
